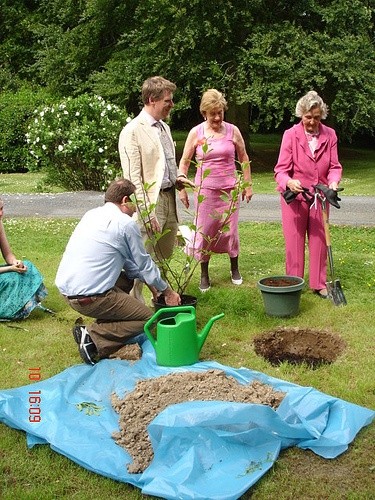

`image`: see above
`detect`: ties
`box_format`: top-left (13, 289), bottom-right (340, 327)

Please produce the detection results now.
top-left (155, 122), bottom-right (177, 185)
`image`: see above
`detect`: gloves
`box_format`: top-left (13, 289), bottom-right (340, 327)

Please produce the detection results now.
top-left (280, 186), bottom-right (314, 205)
top-left (311, 183), bottom-right (345, 209)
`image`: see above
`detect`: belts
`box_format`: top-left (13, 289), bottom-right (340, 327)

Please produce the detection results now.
top-left (63, 288), bottom-right (111, 300)
top-left (159, 186), bottom-right (174, 192)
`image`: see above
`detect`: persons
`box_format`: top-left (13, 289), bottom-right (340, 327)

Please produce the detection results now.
top-left (0, 197), bottom-right (49, 322)
top-left (275, 91), bottom-right (343, 298)
top-left (55, 179), bottom-right (182, 365)
top-left (176, 88), bottom-right (254, 293)
top-left (119, 76), bottom-right (196, 307)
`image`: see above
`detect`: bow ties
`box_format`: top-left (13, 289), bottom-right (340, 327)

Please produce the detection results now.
top-left (305, 132), bottom-right (320, 141)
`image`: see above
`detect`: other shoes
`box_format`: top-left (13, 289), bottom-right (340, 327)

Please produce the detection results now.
top-left (199, 282), bottom-right (211, 294)
top-left (313, 288), bottom-right (332, 300)
top-left (230, 269), bottom-right (243, 285)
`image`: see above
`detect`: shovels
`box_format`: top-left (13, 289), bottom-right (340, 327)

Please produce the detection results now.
top-left (316, 188), bottom-right (347, 308)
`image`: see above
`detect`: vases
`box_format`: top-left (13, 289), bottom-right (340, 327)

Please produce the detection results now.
top-left (258, 275), bottom-right (304, 317)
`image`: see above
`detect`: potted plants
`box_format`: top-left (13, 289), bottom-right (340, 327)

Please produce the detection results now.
top-left (122, 135), bottom-right (252, 325)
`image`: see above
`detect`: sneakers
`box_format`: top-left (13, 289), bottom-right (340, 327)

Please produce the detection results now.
top-left (73, 324), bottom-right (97, 366)
